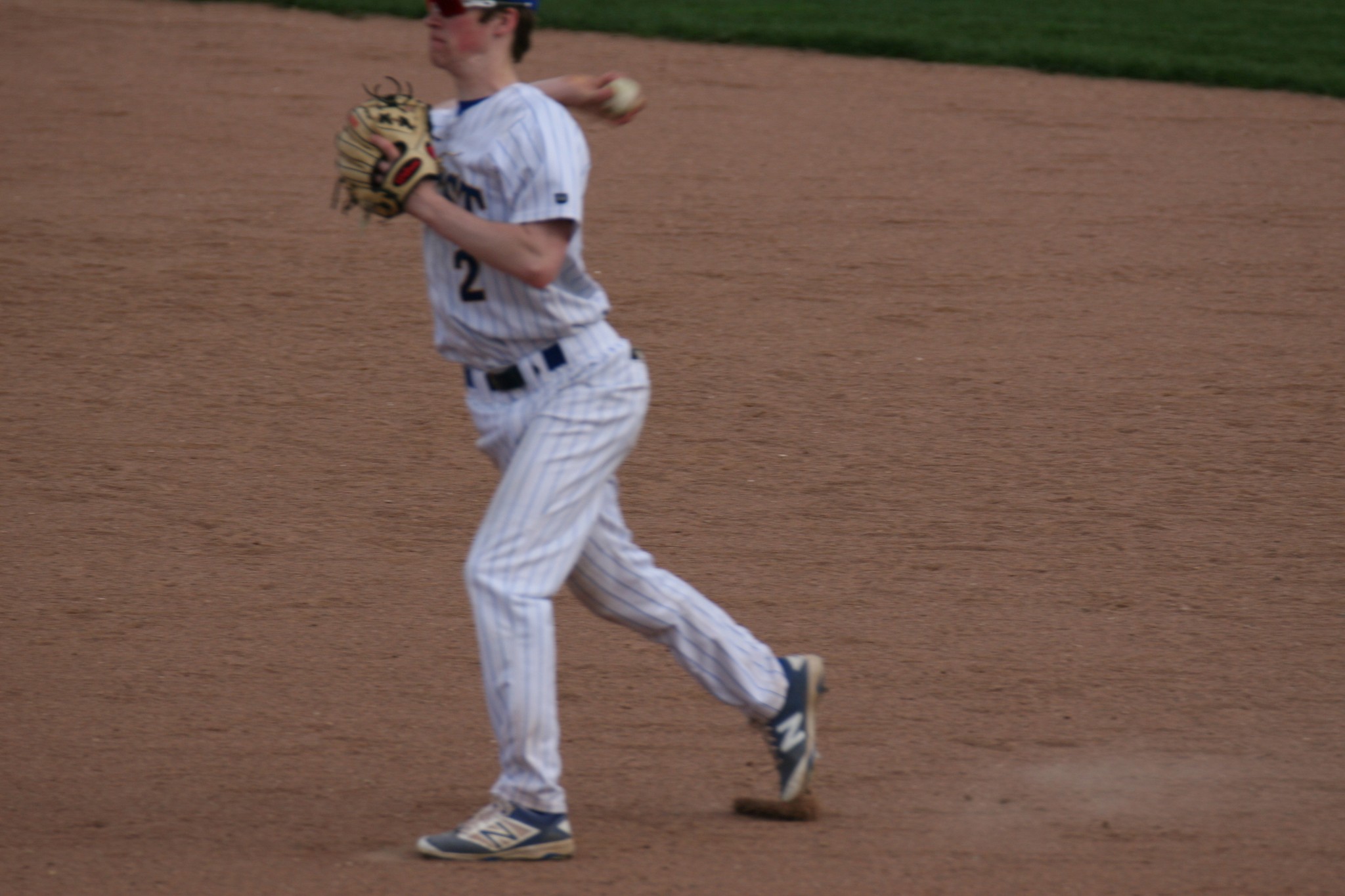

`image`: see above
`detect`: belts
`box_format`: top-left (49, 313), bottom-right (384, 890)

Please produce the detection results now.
top-left (462, 345), bottom-right (567, 393)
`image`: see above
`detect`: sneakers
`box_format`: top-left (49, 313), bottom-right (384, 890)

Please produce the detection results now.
top-left (419, 798), bottom-right (577, 862)
top-left (762, 654), bottom-right (822, 802)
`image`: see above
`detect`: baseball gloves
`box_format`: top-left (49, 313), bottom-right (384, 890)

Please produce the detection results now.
top-left (329, 92), bottom-right (442, 218)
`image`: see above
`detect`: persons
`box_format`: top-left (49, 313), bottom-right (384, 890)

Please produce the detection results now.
top-left (335, 0), bottom-right (830, 862)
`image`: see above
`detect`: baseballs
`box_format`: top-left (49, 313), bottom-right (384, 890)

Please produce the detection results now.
top-left (600, 79), bottom-right (642, 116)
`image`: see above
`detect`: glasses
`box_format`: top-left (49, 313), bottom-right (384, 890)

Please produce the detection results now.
top-left (425, 0), bottom-right (526, 17)
